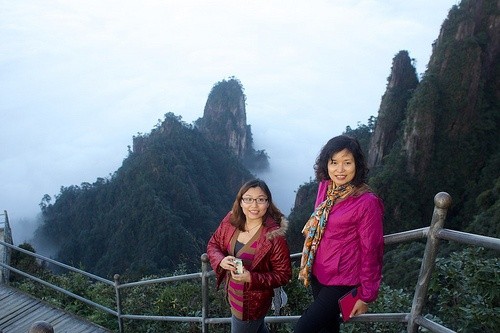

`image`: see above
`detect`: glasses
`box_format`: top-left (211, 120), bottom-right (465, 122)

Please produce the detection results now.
top-left (241, 198), bottom-right (268, 204)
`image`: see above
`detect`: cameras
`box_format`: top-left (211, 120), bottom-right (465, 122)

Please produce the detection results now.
top-left (233, 259), bottom-right (243, 274)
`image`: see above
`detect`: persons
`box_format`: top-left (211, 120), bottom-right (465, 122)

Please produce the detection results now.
top-left (295, 134), bottom-right (385, 333)
top-left (206, 179), bottom-right (292, 333)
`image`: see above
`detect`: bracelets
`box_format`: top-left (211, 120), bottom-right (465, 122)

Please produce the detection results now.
top-left (359, 299), bottom-right (369, 306)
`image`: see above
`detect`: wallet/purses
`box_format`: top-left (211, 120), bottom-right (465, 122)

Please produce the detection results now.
top-left (338, 286), bottom-right (362, 322)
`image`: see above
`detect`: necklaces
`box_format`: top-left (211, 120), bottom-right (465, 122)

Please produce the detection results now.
top-left (245, 220), bottom-right (261, 233)
